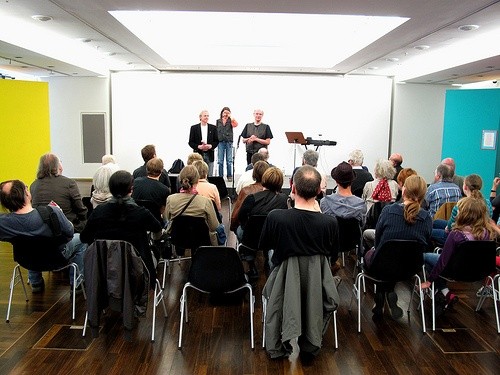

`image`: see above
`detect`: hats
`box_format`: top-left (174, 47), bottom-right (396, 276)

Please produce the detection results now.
top-left (330, 161), bottom-right (357, 183)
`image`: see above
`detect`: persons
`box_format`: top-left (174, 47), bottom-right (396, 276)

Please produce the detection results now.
top-left (0, 180), bottom-right (87, 292)
top-left (414, 157), bottom-right (500, 315)
top-left (133, 144), bottom-right (171, 190)
top-left (240, 108), bottom-right (274, 165)
top-left (164, 152), bottom-right (220, 246)
top-left (83, 155), bottom-right (123, 217)
top-left (229, 148), bottom-right (288, 250)
top-left (363, 175), bottom-right (433, 319)
top-left (304, 149), bottom-right (327, 194)
top-left (360, 153), bottom-right (417, 252)
top-left (189, 110), bottom-right (219, 177)
top-left (261, 167), bottom-right (338, 367)
top-left (79, 170), bottom-right (163, 282)
top-left (215, 107), bottom-right (238, 182)
top-left (132, 157), bottom-right (170, 211)
top-left (30, 153), bottom-right (88, 233)
top-left (320, 161), bottom-right (366, 273)
top-left (347, 150), bottom-right (374, 197)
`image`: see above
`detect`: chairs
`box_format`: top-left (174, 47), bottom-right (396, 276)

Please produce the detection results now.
top-left (207, 176), bottom-right (231, 221)
top-left (361, 202), bottom-right (390, 232)
top-left (336, 215), bottom-right (367, 295)
top-left (178, 245), bottom-right (256, 352)
top-left (82, 239), bottom-right (168, 343)
top-left (236, 214), bottom-right (267, 255)
top-left (260, 254), bottom-right (339, 350)
top-left (161, 215), bottom-right (227, 291)
top-left (6, 237), bottom-right (86, 324)
top-left (417, 240), bottom-right (500, 335)
top-left (348, 239), bottom-right (427, 336)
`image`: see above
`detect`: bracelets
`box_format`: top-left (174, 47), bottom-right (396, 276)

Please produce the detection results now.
top-left (491, 190), bottom-right (496, 192)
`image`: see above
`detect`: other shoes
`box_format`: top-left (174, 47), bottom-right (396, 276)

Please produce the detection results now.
top-left (228, 176), bottom-right (233, 182)
top-left (443, 293), bottom-right (458, 310)
top-left (31, 277), bottom-right (44, 292)
top-left (70, 283), bottom-right (87, 295)
top-left (331, 262), bottom-right (340, 272)
top-left (372, 292), bottom-right (385, 316)
top-left (245, 268), bottom-right (259, 278)
top-left (386, 291), bottom-right (404, 320)
